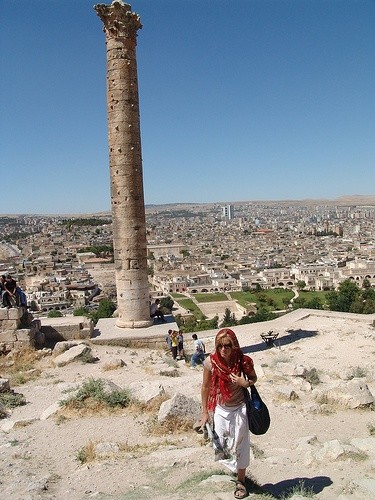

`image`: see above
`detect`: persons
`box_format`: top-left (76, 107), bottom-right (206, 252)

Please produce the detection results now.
top-left (150, 299), bottom-right (167, 322)
top-left (177, 330), bottom-right (184, 356)
top-left (191, 334), bottom-right (206, 369)
top-left (0, 275), bottom-right (31, 309)
top-left (200, 329), bottom-right (257, 499)
top-left (166, 330), bottom-right (173, 357)
top-left (170, 331), bottom-right (179, 362)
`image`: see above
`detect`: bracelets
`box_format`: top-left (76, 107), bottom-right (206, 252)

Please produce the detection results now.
top-left (247, 381), bottom-right (250, 387)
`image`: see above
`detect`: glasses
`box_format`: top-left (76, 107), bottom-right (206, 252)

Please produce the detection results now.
top-left (219, 344), bottom-right (232, 350)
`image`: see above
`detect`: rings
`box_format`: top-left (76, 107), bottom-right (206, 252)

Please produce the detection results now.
top-left (233, 378), bottom-right (235, 380)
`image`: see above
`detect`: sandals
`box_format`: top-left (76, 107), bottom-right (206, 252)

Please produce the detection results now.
top-left (233, 480), bottom-right (248, 498)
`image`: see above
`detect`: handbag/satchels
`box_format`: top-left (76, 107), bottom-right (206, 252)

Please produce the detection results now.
top-left (242, 384), bottom-right (270, 435)
